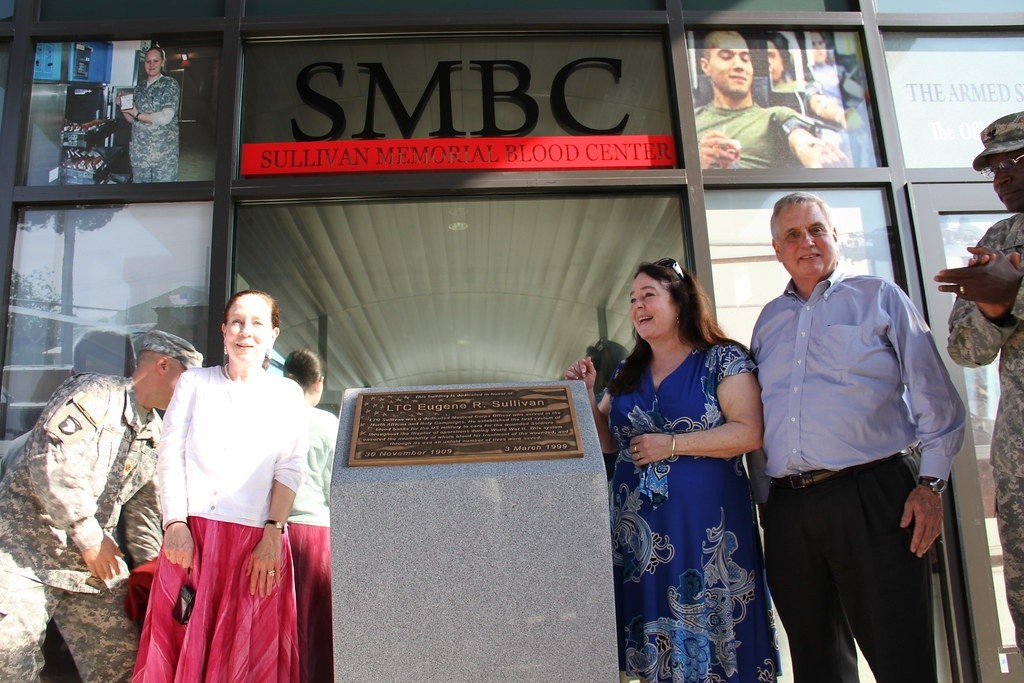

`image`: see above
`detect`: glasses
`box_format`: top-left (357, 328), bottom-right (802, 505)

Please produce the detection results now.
top-left (980, 153), bottom-right (1024, 178)
top-left (657, 256), bottom-right (685, 282)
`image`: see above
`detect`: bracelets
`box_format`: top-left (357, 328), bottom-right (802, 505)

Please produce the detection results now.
top-left (135, 112), bottom-right (141, 121)
top-left (566, 257), bottom-right (783, 683)
top-left (671, 433), bottom-right (676, 457)
top-left (166, 521), bottom-right (189, 528)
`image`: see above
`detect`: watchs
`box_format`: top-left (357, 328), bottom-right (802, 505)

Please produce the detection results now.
top-left (919, 478), bottom-right (947, 493)
top-left (265, 520), bottom-right (284, 534)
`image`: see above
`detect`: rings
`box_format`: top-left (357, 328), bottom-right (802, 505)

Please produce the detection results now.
top-left (959, 285), bottom-right (965, 295)
top-left (634, 444), bottom-right (637, 452)
top-left (638, 452), bottom-right (641, 458)
top-left (267, 569), bottom-right (275, 575)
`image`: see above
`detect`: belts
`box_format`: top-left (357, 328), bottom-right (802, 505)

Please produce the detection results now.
top-left (774, 446), bottom-right (913, 490)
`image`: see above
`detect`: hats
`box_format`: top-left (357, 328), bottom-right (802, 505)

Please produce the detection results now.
top-left (973, 110), bottom-right (1024, 171)
top-left (139, 328), bottom-right (203, 369)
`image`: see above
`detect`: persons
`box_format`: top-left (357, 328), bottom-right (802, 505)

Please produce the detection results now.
top-left (769, 32), bottom-right (864, 133)
top-left (0, 330), bottom-right (203, 683)
top-left (811, 32), bottom-right (869, 109)
top-left (695, 31), bottom-right (850, 170)
top-left (116, 47), bottom-right (180, 182)
top-left (746, 192), bottom-right (966, 683)
top-left (934, 111), bottom-right (1024, 660)
top-left (584, 338), bottom-right (630, 499)
top-left (284, 348), bottom-right (339, 683)
top-left (132, 289), bottom-right (307, 683)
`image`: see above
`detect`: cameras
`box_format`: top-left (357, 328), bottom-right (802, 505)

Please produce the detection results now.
top-left (172, 584), bottom-right (196, 624)
